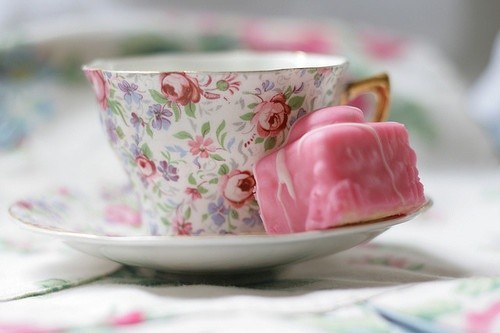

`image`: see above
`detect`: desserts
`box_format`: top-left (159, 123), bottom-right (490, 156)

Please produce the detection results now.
top-left (253, 105), bottom-right (426, 234)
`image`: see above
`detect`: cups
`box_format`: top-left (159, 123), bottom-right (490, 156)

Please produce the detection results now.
top-left (81, 51), bottom-right (393, 234)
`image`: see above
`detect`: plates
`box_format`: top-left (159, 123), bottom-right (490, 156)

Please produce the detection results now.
top-left (10, 175), bottom-right (434, 273)
